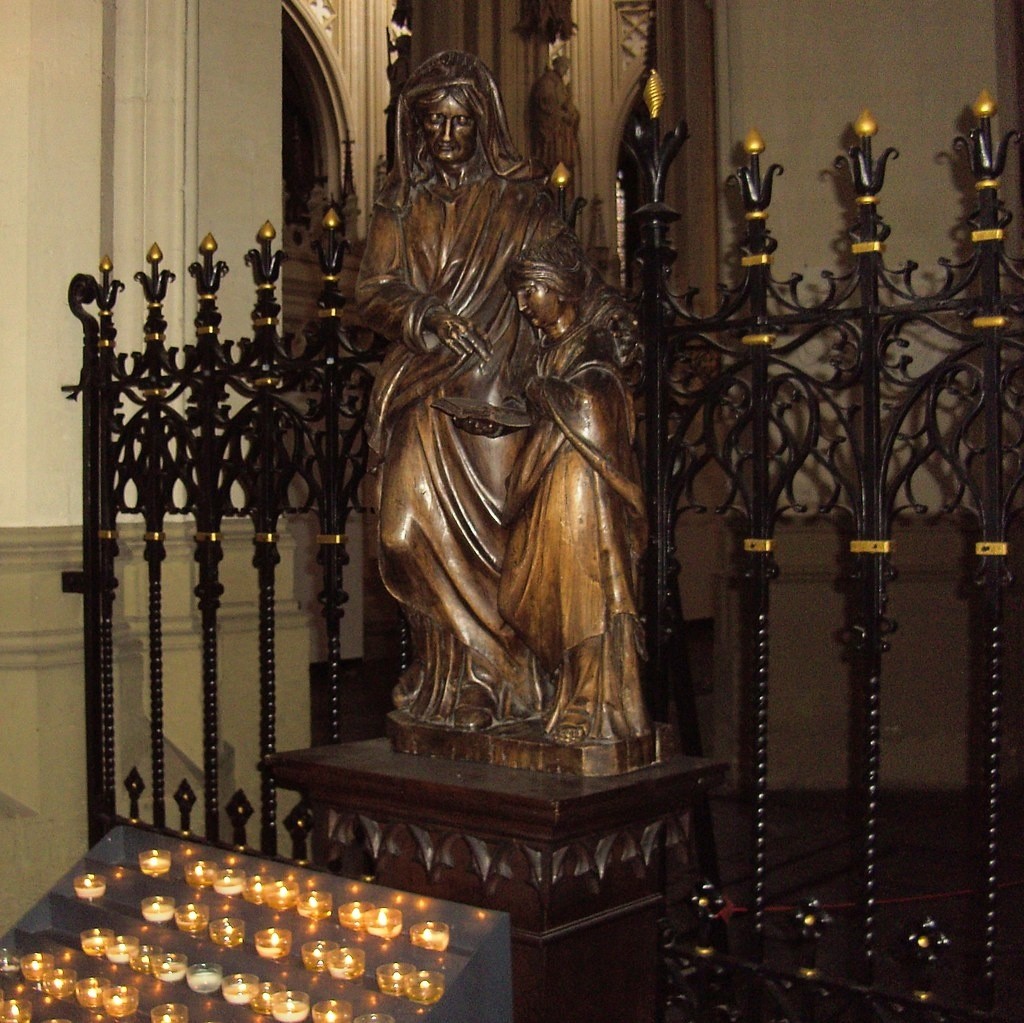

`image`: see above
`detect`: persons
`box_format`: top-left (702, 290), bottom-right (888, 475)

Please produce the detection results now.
top-left (384, 35), bottom-right (412, 169)
top-left (350, 49), bottom-right (651, 745)
top-left (531, 55), bottom-right (577, 169)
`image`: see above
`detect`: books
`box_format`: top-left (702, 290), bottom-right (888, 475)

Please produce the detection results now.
top-left (430, 398), bottom-right (535, 427)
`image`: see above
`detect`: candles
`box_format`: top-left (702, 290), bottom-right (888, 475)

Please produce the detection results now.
top-left (184, 861), bottom-right (218, 890)
top-left (75, 977), bottom-right (111, 1008)
top-left (42, 969), bottom-right (78, 998)
top-left (150, 1003), bottom-right (189, 1023)
top-left (174, 903), bottom-right (209, 933)
top-left (186, 963), bottom-right (223, 994)
top-left (0, 999), bottom-right (32, 1023)
top-left (0, 946), bottom-right (23, 977)
top-left (20, 952), bottom-right (54, 985)
top-left (102, 986), bottom-right (139, 1018)
top-left (103, 936), bottom-right (140, 964)
top-left (139, 850), bottom-right (172, 878)
top-left (129, 945), bottom-right (163, 976)
top-left (80, 929), bottom-right (115, 957)
top-left (141, 896), bottom-right (174, 924)
top-left (150, 952), bottom-right (188, 984)
top-left (208, 868), bottom-right (451, 1023)
top-left (73, 873), bottom-right (106, 899)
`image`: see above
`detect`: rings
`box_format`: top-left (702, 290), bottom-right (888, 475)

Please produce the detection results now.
top-left (448, 326), bottom-right (456, 337)
